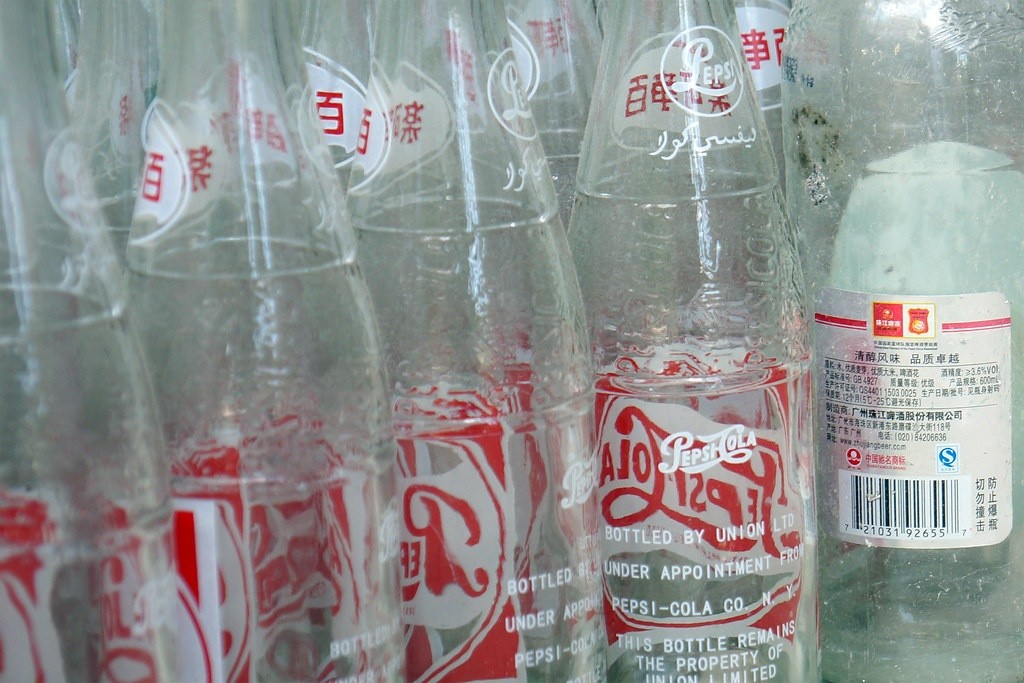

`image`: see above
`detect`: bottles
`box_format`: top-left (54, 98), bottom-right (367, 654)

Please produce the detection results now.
top-left (0, 0), bottom-right (1024, 683)
